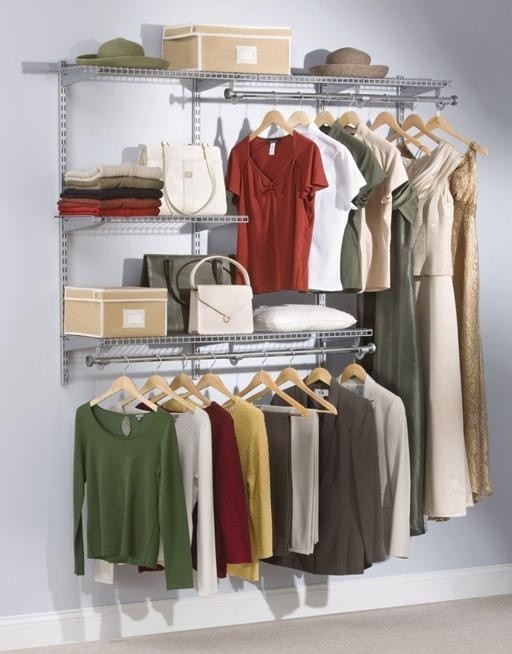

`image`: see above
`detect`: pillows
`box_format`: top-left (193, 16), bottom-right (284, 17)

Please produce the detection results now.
top-left (254, 304), bottom-right (356, 333)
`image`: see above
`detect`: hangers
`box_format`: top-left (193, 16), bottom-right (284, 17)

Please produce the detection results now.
top-left (240, 93), bottom-right (486, 167)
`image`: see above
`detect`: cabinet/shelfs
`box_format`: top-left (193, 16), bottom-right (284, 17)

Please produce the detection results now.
top-left (22, 61), bottom-right (458, 386)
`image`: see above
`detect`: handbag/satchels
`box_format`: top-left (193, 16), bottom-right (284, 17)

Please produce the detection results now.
top-left (140, 255), bottom-right (235, 334)
top-left (188, 255), bottom-right (254, 335)
top-left (138, 141), bottom-right (228, 217)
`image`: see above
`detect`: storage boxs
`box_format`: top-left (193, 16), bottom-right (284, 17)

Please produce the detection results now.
top-left (161, 24), bottom-right (291, 74)
top-left (62, 286), bottom-right (167, 339)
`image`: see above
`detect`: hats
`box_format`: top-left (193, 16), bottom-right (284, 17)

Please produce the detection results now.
top-left (75, 38), bottom-right (170, 69)
top-left (309, 46), bottom-right (389, 78)
